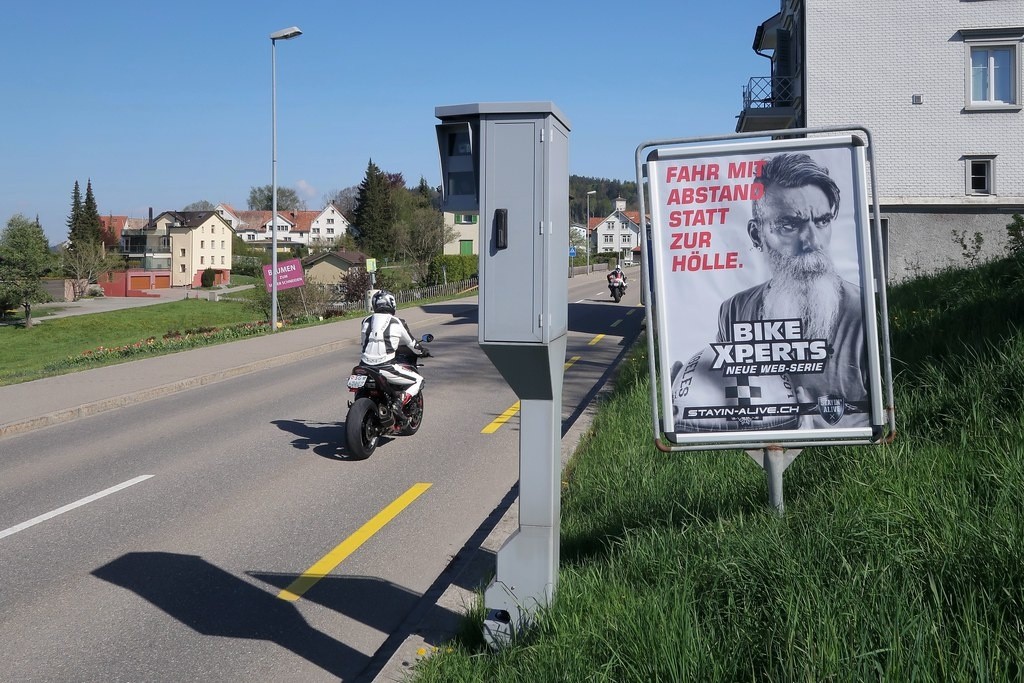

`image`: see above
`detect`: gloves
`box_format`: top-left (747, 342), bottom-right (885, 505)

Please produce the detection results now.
top-left (420, 348), bottom-right (429, 358)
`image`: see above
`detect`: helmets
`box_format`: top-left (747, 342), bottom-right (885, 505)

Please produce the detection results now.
top-left (615, 265), bottom-right (620, 270)
top-left (372, 290), bottom-right (396, 315)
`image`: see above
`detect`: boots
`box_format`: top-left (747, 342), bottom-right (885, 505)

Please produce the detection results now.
top-left (390, 391), bottom-right (413, 420)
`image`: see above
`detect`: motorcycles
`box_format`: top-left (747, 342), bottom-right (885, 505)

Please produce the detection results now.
top-left (608, 277), bottom-right (628, 303)
top-left (346, 334), bottom-right (434, 459)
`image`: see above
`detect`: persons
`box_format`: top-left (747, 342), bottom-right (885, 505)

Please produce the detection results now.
top-left (668, 153), bottom-right (871, 429)
top-left (606, 265), bottom-right (625, 297)
top-left (360, 293), bottom-right (430, 421)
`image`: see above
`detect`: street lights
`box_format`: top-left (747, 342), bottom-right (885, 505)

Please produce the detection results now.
top-left (587, 191), bottom-right (596, 275)
top-left (270, 26), bottom-right (304, 332)
